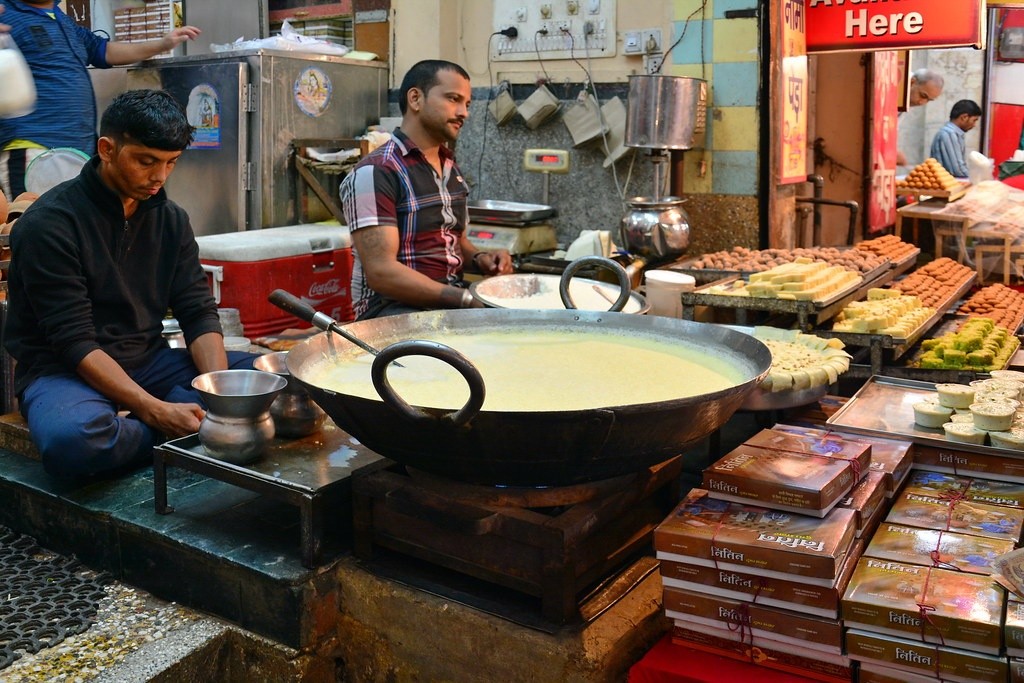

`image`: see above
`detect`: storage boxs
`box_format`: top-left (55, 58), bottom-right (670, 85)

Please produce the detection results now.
top-left (653, 424), bottom-right (1024, 683)
top-left (196, 222), bottom-right (357, 343)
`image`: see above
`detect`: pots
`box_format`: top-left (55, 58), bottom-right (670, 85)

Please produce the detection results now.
top-left (281, 257), bottom-right (774, 486)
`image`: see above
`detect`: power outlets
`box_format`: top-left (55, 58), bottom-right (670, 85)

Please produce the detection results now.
top-left (567, 0), bottom-right (579, 16)
top-left (643, 29), bottom-right (662, 54)
top-left (642, 55), bottom-right (665, 77)
top-left (516, 6), bottom-right (526, 22)
top-left (540, 20), bottom-right (572, 37)
top-left (589, 0), bottom-right (601, 14)
top-left (540, 3), bottom-right (552, 19)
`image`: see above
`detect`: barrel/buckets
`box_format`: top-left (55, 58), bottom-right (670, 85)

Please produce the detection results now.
top-left (623, 74), bottom-right (708, 150)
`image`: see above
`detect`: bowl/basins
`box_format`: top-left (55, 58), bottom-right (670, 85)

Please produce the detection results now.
top-left (468, 273), bottom-right (652, 314)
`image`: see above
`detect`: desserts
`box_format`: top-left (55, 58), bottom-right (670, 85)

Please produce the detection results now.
top-left (913, 371), bottom-right (1024, 452)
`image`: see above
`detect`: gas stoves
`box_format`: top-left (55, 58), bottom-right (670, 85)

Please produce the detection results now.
top-left (346, 454), bottom-right (688, 621)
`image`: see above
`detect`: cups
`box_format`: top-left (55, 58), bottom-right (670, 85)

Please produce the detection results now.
top-left (191, 369), bottom-right (288, 465)
top-left (218, 308), bottom-right (251, 352)
top-left (0, 27), bottom-right (37, 120)
top-left (644, 269), bottom-right (696, 318)
top-left (252, 350), bottom-right (327, 439)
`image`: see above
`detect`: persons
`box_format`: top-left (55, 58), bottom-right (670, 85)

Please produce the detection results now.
top-left (896, 70), bottom-right (944, 169)
top-left (0, 89), bottom-right (265, 474)
top-left (930, 99), bottom-right (982, 177)
top-left (0, 0), bottom-right (202, 224)
top-left (339, 59), bottom-right (513, 322)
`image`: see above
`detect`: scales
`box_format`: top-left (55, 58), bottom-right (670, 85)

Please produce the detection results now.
top-left (466, 149), bottom-right (569, 256)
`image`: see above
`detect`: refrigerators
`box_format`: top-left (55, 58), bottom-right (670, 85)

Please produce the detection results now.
top-left (124, 48), bottom-right (388, 237)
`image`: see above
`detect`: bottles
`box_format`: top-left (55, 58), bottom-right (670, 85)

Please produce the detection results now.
top-left (621, 196), bottom-right (691, 265)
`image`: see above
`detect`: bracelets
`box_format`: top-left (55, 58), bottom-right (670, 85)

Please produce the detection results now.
top-left (472, 251), bottom-right (487, 272)
top-left (461, 288), bottom-right (474, 308)
